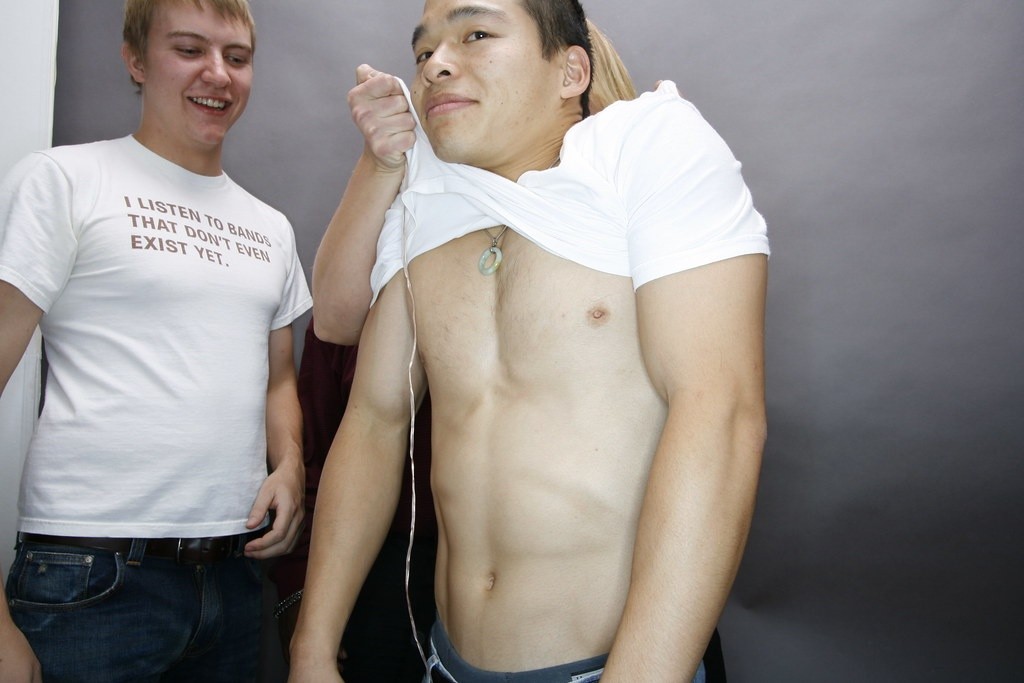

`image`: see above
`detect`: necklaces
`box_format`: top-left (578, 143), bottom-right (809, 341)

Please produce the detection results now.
top-left (478, 155), bottom-right (560, 276)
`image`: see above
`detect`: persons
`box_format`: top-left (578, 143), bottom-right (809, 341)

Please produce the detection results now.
top-left (288, 1), bottom-right (770, 683)
top-left (0, 0), bottom-right (314, 683)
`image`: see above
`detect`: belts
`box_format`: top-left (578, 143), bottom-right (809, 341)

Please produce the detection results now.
top-left (16, 528), bottom-right (255, 563)
top-left (424, 636), bottom-right (454, 683)
